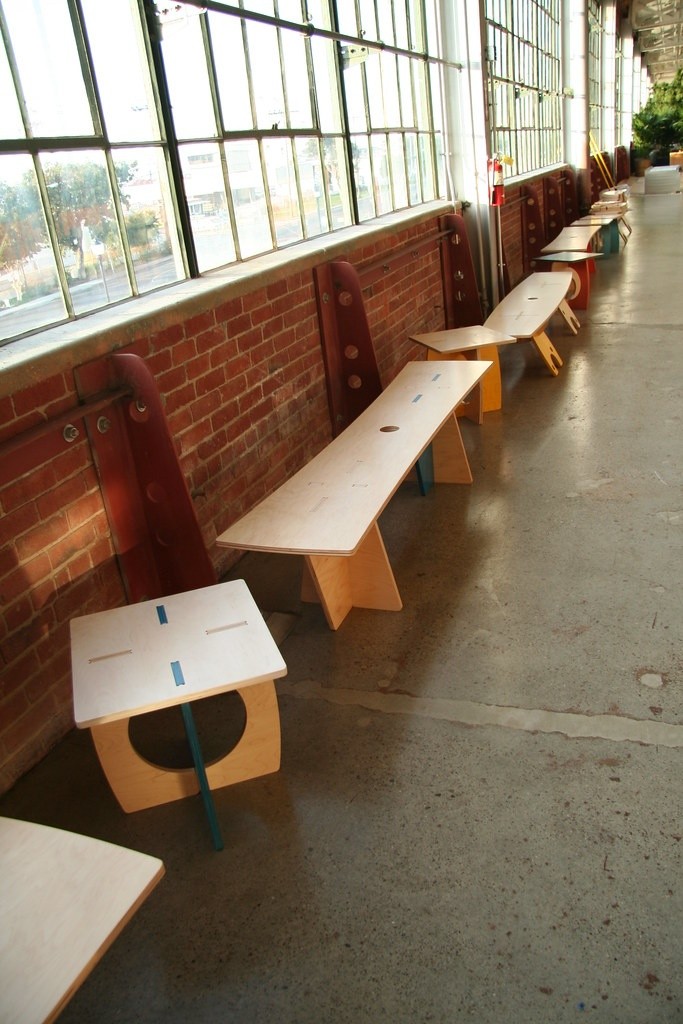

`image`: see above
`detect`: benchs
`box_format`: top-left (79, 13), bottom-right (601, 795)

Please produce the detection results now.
top-left (216, 361), bottom-right (493, 629)
top-left (540, 226), bottom-right (601, 310)
top-left (484, 272), bottom-right (580, 377)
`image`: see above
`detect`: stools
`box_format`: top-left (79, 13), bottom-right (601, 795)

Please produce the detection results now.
top-left (71, 579), bottom-right (289, 813)
top-left (580, 214), bottom-right (631, 243)
top-left (408, 326), bottom-right (517, 424)
top-left (570, 219), bottom-right (619, 259)
top-left (532, 251), bottom-right (603, 301)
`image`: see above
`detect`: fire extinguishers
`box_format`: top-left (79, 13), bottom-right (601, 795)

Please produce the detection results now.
top-left (486, 152), bottom-right (514, 207)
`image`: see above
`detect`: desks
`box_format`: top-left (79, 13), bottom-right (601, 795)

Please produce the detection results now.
top-left (0, 815), bottom-right (167, 1023)
